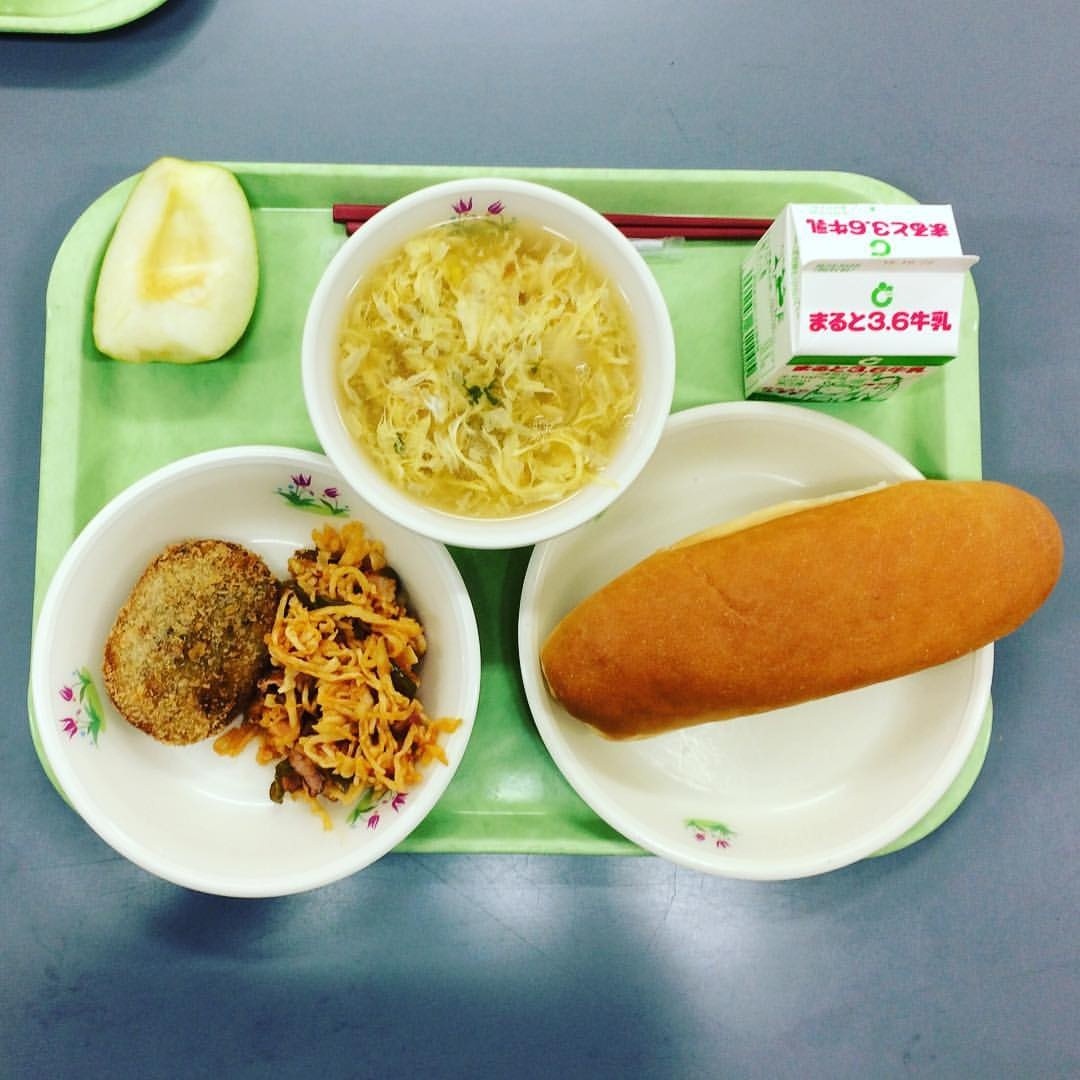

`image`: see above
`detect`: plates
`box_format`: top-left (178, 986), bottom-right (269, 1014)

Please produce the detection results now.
top-left (519, 400), bottom-right (995, 881)
top-left (32, 445), bottom-right (481, 897)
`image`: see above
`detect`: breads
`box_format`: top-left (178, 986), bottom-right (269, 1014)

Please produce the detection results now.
top-left (542, 481), bottom-right (1065, 743)
top-left (103, 538), bottom-right (280, 745)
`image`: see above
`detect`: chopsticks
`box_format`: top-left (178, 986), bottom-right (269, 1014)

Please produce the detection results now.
top-left (332, 203), bottom-right (778, 241)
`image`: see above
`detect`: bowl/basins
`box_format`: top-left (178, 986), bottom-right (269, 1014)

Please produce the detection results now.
top-left (301, 176), bottom-right (677, 551)
